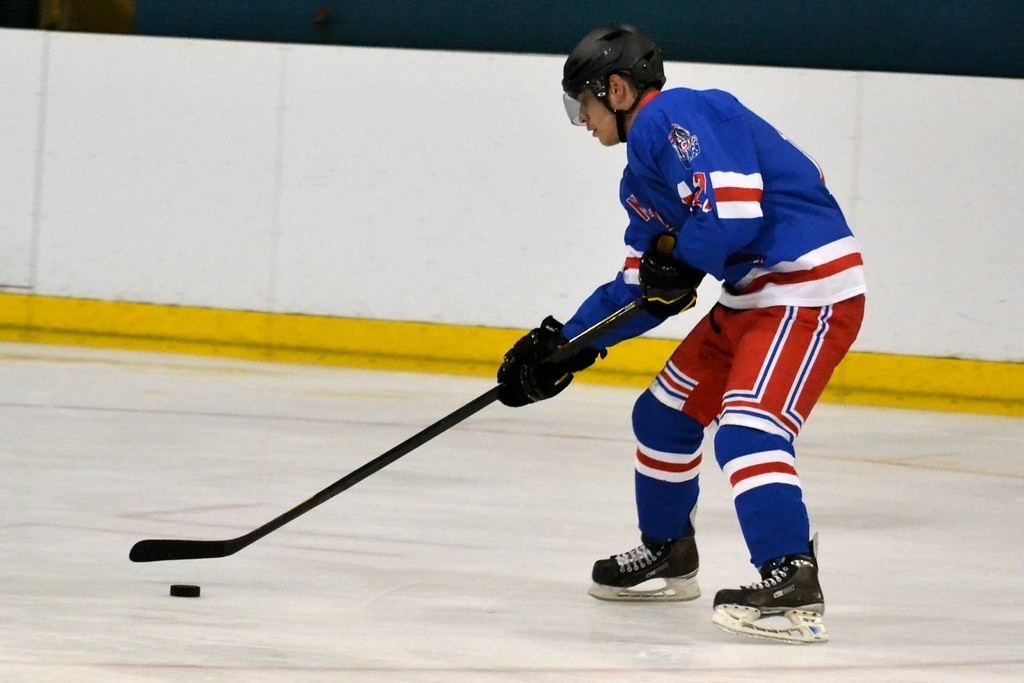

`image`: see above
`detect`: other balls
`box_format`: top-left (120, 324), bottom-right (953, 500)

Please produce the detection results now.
top-left (169, 583), bottom-right (201, 599)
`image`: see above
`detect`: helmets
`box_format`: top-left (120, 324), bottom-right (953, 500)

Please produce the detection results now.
top-left (562, 23), bottom-right (667, 101)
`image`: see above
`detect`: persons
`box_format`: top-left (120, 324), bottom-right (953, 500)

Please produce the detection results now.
top-left (497, 21), bottom-right (868, 646)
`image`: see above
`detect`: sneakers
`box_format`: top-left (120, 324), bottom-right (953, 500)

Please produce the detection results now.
top-left (710, 532), bottom-right (828, 645)
top-left (587, 534), bottom-right (699, 603)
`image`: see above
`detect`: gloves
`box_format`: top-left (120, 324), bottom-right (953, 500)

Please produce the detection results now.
top-left (495, 315), bottom-right (600, 408)
top-left (637, 230), bottom-right (704, 317)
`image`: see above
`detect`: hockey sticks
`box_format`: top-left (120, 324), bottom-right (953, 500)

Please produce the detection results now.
top-left (128, 297), bottom-right (646, 565)
